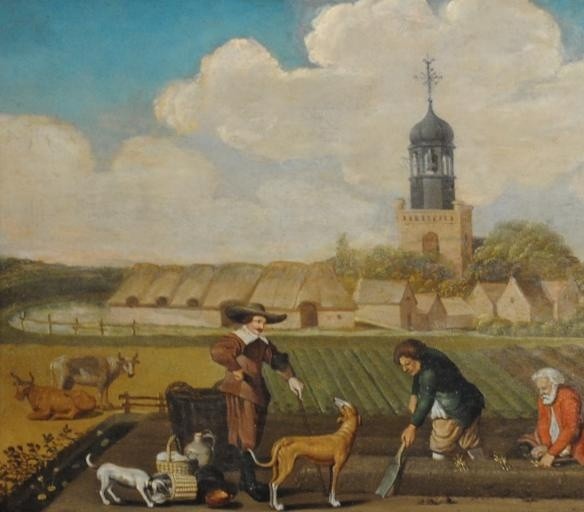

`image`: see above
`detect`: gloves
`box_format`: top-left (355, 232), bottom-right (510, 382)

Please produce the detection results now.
top-left (288, 377), bottom-right (304, 399)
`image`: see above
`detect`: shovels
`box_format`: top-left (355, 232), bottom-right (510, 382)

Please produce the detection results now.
top-left (374, 439), bottom-right (406, 498)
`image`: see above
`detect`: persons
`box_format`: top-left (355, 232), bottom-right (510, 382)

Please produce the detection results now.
top-left (517, 368), bottom-right (583, 469)
top-left (393, 337), bottom-right (486, 459)
top-left (210, 303), bottom-right (304, 501)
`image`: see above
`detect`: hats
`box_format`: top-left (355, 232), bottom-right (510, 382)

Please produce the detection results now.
top-left (225, 303), bottom-right (286, 323)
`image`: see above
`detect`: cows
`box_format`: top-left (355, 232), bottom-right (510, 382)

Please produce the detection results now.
top-left (7, 369), bottom-right (100, 421)
top-left (47, 348), bottom-right (142, 413)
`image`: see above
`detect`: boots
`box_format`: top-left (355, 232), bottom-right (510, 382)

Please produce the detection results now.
top-left (239, 451), bottom-right (269, 501)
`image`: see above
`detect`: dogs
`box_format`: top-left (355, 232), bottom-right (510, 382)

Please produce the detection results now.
top-left (246, 396), bottom-right (363, 511)
top-left (84, 452), bottom-right (172, 509)
